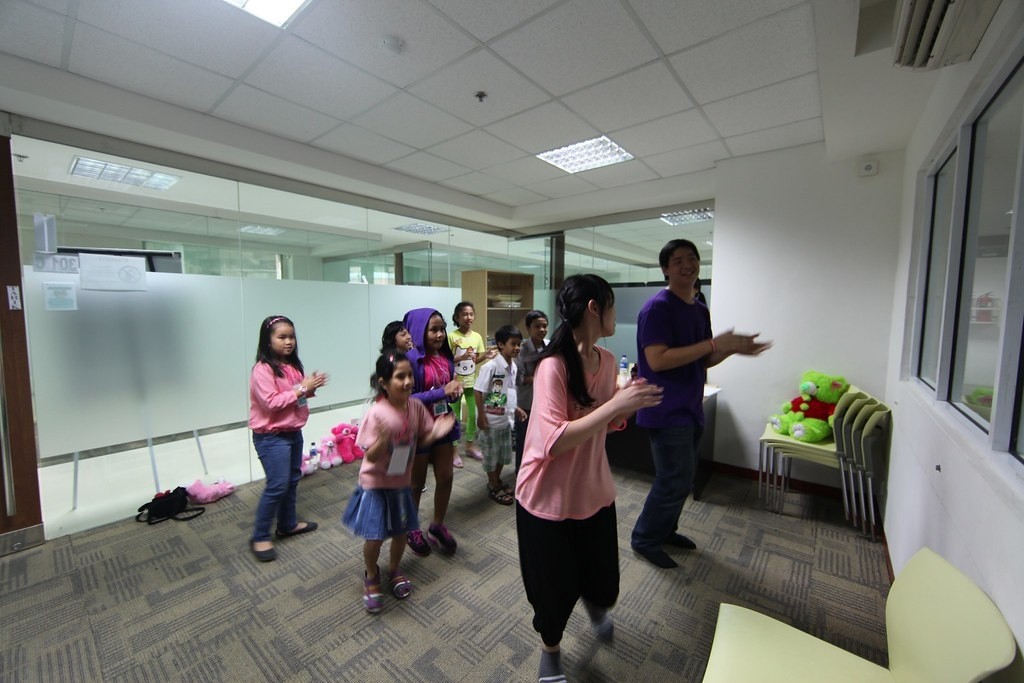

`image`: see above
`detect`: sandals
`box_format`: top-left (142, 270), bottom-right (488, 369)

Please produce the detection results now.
top-left (487, 479), bottom-right (514, 505)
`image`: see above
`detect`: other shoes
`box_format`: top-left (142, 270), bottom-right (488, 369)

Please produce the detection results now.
top-left (249, 538), bottom-right (278, 562)
top-left (274, 521), bottom-right (318, 539)
top-left (452, 456), bottom-right (464, 468)
top-left (362, 563), bottom-right (385, 612)
top-left (465, 450), bottom-right (483, 459)
top-left (386, 569), bottom-right (413, 598)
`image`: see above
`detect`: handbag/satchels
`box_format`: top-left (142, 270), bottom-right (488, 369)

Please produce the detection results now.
top-left (135, 486), bottom-right (205, 526)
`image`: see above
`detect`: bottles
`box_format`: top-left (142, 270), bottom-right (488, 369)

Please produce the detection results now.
top-left (619, 355), bottom-right (628, 383)
top-left (310, 442), bottom-right (318, 470)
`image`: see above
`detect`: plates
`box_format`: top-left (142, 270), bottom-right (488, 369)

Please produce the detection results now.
top-left (491, 301), bottom-right (522, 307)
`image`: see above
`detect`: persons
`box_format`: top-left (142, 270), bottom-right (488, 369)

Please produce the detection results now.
top-left (380, 321), bottom-right (415, 356)
top-left (473, 325), bottom-right (529, 506)
top-left (341, 351), bottom-right (456, 613)
top-left (514, 309), bottom-right (549, 476)
top-left (692, 277), bottom-right (707, 305)
top-left (446, 301), bottom-right (498, 468)
top-left (631, 238), bottom-right (775, 569)
top-left (400, 307), bottom-right (465, 556)
top-left (245, 316), bottom-right (328, 563)
top-left (515, 275), bottom-right (664, 683)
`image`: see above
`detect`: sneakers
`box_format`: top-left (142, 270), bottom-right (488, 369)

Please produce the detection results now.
top-left (406, 529), bottom-right (432, 556)
top-left (427, 522), bottom-right (457, 553)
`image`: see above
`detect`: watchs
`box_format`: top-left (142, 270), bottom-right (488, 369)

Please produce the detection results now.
top-left (294, 383), bottom-right (308, 396)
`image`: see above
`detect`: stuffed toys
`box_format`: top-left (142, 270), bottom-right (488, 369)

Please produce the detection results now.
top-left (768, 370), bottom-right (850, 443)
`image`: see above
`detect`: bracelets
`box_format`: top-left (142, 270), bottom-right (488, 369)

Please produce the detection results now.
top-left (709, 338), bottom-right (717, 353)
top-left (610, 417), bottom-right (627, 431)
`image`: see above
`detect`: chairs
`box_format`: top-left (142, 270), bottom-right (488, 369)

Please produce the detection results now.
top-left (701, 546), bottom-right (1017, 683)
top-left (756, 383), bottom-right (891, 545)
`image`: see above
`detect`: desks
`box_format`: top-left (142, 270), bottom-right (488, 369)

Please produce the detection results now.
top-left (604, 374), bottom-right (721, 502)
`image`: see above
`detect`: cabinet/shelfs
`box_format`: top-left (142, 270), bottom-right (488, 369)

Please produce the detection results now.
top-left (461, 269), bottom-right (535, 423)
